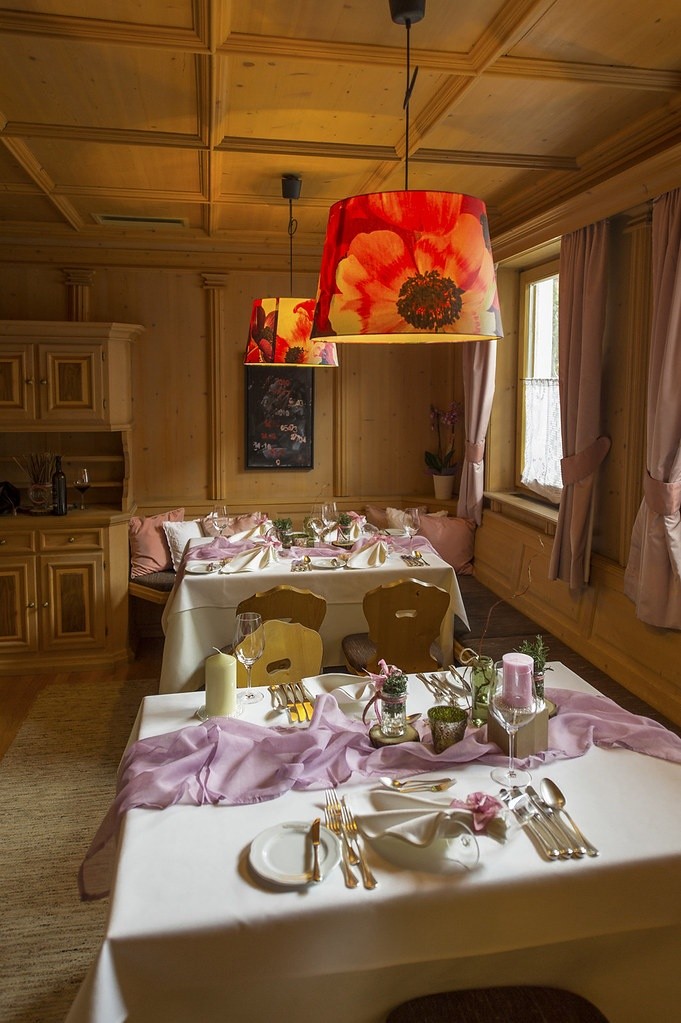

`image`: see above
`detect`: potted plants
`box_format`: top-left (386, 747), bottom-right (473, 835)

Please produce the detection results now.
top-left (275, 518), bottom-right (294, 549)
top-left (513, 634), bottom-right (554, 701)
top-left (338, 512), bottom-right (351, 543)
top-left (381, 671), bottom-right (408, 737)
top-left (427, 705), bottom-right (468, 754)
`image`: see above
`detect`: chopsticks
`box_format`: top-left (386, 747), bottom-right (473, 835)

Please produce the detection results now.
top-left (10, 448), bottom-right (58, 482)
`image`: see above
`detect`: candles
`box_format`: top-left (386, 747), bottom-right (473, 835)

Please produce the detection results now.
top-left (504, 652), bottom-right (534, 708)
top-left (206, 647), bottom-right (237, 716)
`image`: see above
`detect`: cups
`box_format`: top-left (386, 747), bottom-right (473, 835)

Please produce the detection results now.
top-left (427, 706), bottom-right (469, 753)
top-left (30, 482), bottom-right (52, 515)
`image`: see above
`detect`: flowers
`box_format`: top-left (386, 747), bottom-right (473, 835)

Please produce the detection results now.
top-left (424, 399), bottom-right (464, 475)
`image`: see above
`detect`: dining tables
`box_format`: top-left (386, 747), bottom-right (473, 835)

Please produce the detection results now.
top-left (159, 531), bottom-right (471, 694)
top-left (65, 661), bottom-right (681, 1023)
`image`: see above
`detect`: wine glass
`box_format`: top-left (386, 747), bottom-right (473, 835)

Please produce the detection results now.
top-left (233, 612), bottom-right (265, 703)
top-left (304, 500), bottom-right (339, 548)
top-left (488, 661), bottom-right (537, 786)
top-left (212, 504), bottom-right (229, 537)
top-left (74, 467), bottom-right (91, 512)
top-left (403, 508), bottom-right (420, 545)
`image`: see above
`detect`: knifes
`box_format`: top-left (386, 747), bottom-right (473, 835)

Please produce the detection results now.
top-left (311, 817), bottom-right (322, 882)
top-left (282, 680), bottom-right (314, 722)
top-left (401, 555), bottom-right (424, 566)
top-left (500, 785), bottom-right (586, 860)
top-left (448, 665), bottom-right (472, 692)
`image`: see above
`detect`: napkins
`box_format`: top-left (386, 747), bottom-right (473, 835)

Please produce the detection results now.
top-left (348, 540), bottom-right (389, 570)
top-left (354, 789), bottom-right (518, 848)
top-left (228, 520), bottom-right (278, 543)
top-left (219, 543), bottom-right (280, 574)
top-left (324, 520), bottom-right (365, 540)
top-left (298, 673), bottom-right (383, 706)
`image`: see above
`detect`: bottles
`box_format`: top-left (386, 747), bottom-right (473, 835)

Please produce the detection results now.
top-left (51, 456), bottom-right (67, 516)
top-left (458, 648), bottom-right (495, 729)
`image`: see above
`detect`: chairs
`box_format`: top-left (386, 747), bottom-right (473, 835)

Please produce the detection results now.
top-left (196, 578), bottom-right (451, 691)
top-left (385, 983), bottom-right (608, 1023)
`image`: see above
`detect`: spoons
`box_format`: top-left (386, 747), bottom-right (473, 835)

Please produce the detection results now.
top-left (378, 777), bottom-right (451, 790)
top-left (268, 685), bottom-right (288, 714)
top-left (412, 550), bottom-right (431, 566)
top-left (540, 778), bottom-right (598, 856)
top-left (406, 713), bottom-right (422, 725)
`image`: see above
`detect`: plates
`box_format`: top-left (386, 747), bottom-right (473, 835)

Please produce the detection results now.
top-left (380, 528), bottom-right (405, 537)
top-left (447, 666), bottom-right (503, 692)
top-left (312, 557), bottom-right (346, 568)
top-left (185, 563), bottom-right (221, 574)
top-left (249, 821), bottom-right (341, 885)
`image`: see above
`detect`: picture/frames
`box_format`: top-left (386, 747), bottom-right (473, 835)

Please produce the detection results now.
top-left (245, 365), bottom-right (315, 470)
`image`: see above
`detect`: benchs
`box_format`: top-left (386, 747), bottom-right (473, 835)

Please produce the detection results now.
top-left (129, 497), bottom-right (681, 739)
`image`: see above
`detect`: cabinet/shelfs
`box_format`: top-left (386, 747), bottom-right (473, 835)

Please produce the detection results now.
top-left (0, 320), bottom-right (147, 676)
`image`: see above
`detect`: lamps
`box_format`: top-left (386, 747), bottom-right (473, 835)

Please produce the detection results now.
top-left (242, 173), bottom-right (339, 367)
top-left (309, 0), bottom-right (504, 344)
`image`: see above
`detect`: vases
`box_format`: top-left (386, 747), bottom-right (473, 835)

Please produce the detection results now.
top-left (433, 474), bottom-right (454, 500)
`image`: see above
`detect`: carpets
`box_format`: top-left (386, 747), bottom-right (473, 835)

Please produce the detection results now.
top-left (0, 679), bottom-right (159, 1023)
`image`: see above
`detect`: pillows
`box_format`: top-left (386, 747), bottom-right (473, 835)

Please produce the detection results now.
top-left (128, 505), bottom-right (477, 578)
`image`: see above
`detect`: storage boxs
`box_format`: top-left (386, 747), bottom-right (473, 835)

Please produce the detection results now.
top-left (487, 706), bottom-right (548, 759)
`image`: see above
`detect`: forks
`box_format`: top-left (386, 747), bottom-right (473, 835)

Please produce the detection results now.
top-left (400, 778), bottom-right (457, 792)
top-left (324, 789), bottom-right (378, 889)
